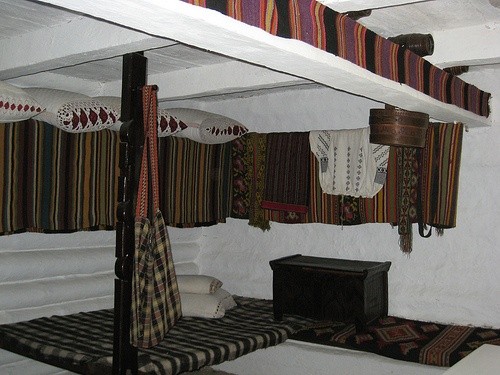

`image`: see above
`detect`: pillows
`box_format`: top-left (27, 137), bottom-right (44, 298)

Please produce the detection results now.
top-left (93, 95), bottom-right (188, 138)
top-left (178, 289), bottom-right (237, 319)
top-left (21, 87), bottom-right (120, 133)
top-left (176, 275), bottom-right (223, 294)
top-left (164, 108), bottom-right (248, 145)
top-left (0, 82), bottom-right (46, 124)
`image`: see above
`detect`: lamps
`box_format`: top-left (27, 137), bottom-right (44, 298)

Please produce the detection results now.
top-left (388, 33), bottom-right (434, 57)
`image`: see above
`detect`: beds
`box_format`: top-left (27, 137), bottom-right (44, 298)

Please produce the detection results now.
top-left (0, 295), bottom-right (492, 375)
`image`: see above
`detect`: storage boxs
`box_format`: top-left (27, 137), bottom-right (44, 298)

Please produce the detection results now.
top-left (268, 254), bottom-right (391, 331)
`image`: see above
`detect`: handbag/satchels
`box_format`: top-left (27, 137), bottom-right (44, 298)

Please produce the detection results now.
top-left (129, 83), bottom-right (182, 350)
